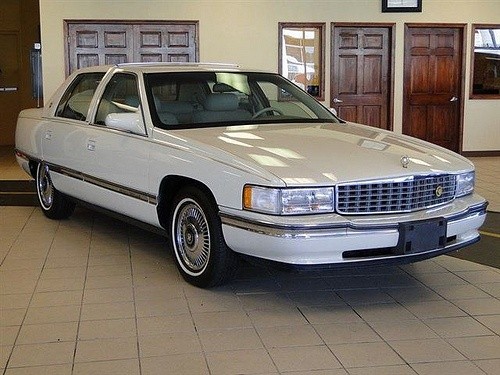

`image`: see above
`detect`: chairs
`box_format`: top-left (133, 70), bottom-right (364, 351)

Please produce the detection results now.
top-left (193, 93), bottom-right (252, 122)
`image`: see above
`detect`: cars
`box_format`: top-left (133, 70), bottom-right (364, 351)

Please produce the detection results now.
top-left (13, 62), bottom-right (490, 290)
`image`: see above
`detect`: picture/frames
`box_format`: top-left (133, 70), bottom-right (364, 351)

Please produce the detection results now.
top-left (382, 0), bottom-right (422, 12)
